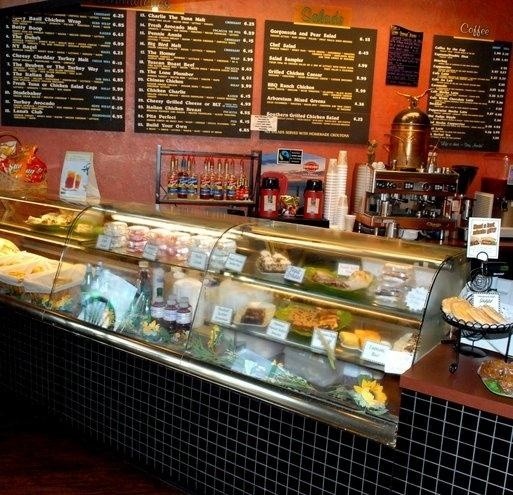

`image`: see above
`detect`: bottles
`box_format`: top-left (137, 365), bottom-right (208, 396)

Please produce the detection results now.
top-left (258, 177), bottom-right (280, 218)
top-left (151, 297), bottom-right (166, 324)
top-left (187, 157), bottom-right (198, 200)
top-left (214, 159), bottom-right (224, 200)
top-left (209, 158), bottom-right (216, 199)
top-left (200, 157), bottom-right (210, 199)
top-left (226, 159), bottom-right (237, 200)
top-left (165, 294), bottom-right (179, 309)
top-left (169, 155), bottom-right (178, 200)
top-left (178, 296), bottom-right (192, 313)
top-left (178, 156), bottom-right (189, 198)
top-left (80, 263), bottom-right (95, 302)
top-left (92, 267), bottom-right (102, 287)
top-left (163, 300), bottom-right (177, 334)
top-left (223, 159), bottom-right (230, 200)
top-left (238, 160), bottom-right (245, 186)
top-left (303, 179), bottom-right (324, 219)
top-left (175, 302), bottom-right (192, 332)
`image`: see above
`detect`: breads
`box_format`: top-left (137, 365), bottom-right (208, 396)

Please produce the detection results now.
top-left (339, 332), bottom-right (358, 348)
top-left (354, 329), bottom-right (381, 349)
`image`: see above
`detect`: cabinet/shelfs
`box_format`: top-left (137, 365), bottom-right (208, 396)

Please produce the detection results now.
top-left (350, 161), bottom-right (459, 246)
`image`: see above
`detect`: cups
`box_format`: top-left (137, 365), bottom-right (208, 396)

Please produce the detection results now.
top-left (324, 151), bottom-right (355, 232)
top-left (354, 165), bottom-right (373, 217)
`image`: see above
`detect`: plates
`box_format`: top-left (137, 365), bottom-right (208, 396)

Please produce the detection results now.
top-left (302, 268), bottom-right (374, 300)
top-left (275, 300), bottom-right (354, 337)
top-left (26, 222), bottom-right (75, 235)
top-left (481, 360), bottom-right (513, 400)
top-left (437, 296), bottom-right (513, 328)
